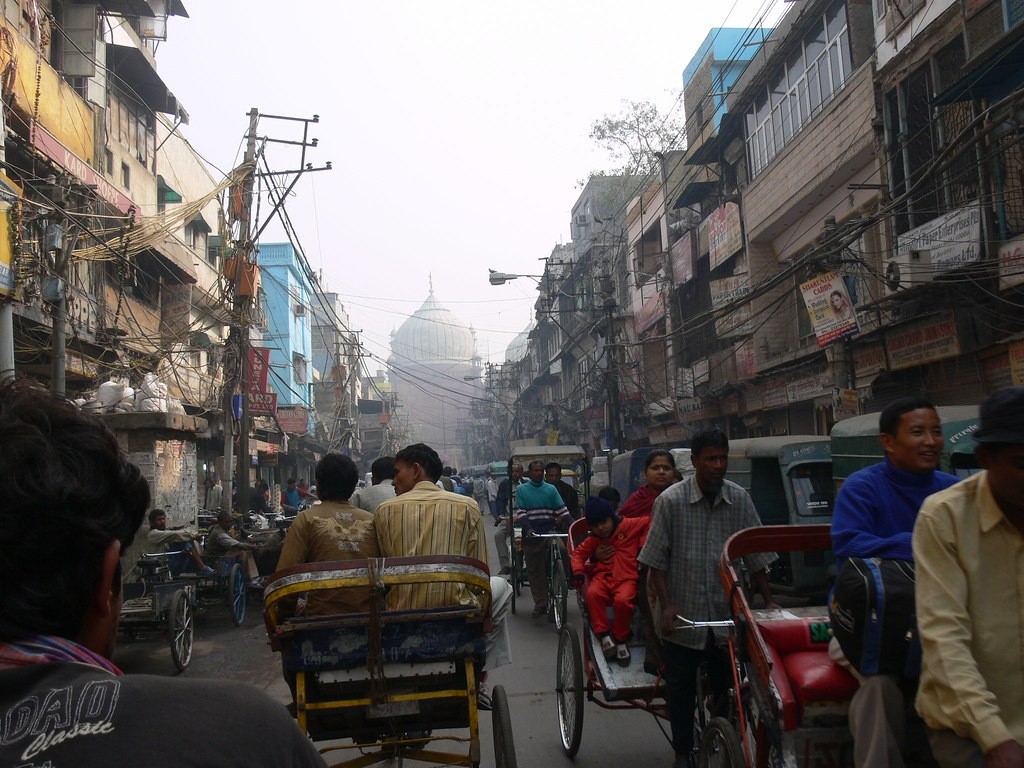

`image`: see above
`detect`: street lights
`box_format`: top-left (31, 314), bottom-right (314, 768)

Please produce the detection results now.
top-left (488, 272), bottom-right (623, 455)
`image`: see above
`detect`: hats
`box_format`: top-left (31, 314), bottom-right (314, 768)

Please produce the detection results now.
top-left (972, 386), bottom-right (1024, 443)
top-left (586, 494), bottom-right (611, 519)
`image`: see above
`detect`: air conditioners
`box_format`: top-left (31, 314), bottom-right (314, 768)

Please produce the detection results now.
top-left (575, 214), bottom-right (590, 227)
top-left (883, 249), bottom-right (933, 297)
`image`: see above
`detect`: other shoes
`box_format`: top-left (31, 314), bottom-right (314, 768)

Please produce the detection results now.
top-left (602, 635), bottom-right (633, 667)
top-left (532, 605), bottom-right (546, 618)
top-left (499, 567), bottom-right (510, 575)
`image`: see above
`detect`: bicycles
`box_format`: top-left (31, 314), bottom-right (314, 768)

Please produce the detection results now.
top-left (499, 514), bottom-right (521, 615)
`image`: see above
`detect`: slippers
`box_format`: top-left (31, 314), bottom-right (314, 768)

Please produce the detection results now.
top-left (246, 577), bottom-right (265, 589)
top-left (198, 566), bottom-right (217, 574)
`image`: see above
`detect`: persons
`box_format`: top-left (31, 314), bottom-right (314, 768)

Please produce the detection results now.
top-left (205, 511), bottom-right (268, 590)
top-left (494, 460), bottom-right (581, 619)
top-left (911, 386), bottom-right (1024, 768)
top-left (827, 395), bottom-right (965, 768)
top-left (349, 456), bottom-right (397, 515)
top-left (438, 466), bottom-right (499, 517)
top-left (374, 443), bottom-right (513, 711)
top-left (275, 452), bottom-right (382, 721)
top-left (203, 477), bottom-right (319, 518)
top-left (145, 509), bottom-right (218, 578)
top-left (0, 368), bottom-right (330, 768)
top-left (570, 429), bottom-right (763, 768)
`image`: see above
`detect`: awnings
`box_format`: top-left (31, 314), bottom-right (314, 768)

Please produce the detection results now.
top-left (854, 266), bottom-right (985, 313)
top-left (29, 117), bottom-right (142, 226)
top-left (673, 181), bottom-right (718, 209)
top-left (126, 213), bottom-right (198, 285)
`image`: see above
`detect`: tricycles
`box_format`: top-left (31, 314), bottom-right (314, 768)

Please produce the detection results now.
top-left (263, 554), bottom-right (517, 767)
top-left (508, 446), bottom-right (591, 628)
top-left (117, 550), bottom-right (196, 671)
top-left (197, 556), bottom-right (247, 627)
top-left (460, 461), bottom-right (508, 487)
top-left (554, 516), bottom-right (748, 768)
top-left (696, 525), bottom-right (919, 767)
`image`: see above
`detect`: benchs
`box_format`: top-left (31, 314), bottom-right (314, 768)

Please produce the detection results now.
top-left (757, 616), bottom-right (861, 732)
top-left (277, 605), bottom-right (486, 678)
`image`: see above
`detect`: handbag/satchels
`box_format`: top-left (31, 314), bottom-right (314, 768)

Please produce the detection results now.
top-left (830, 559), bottom-right (920, 675)
